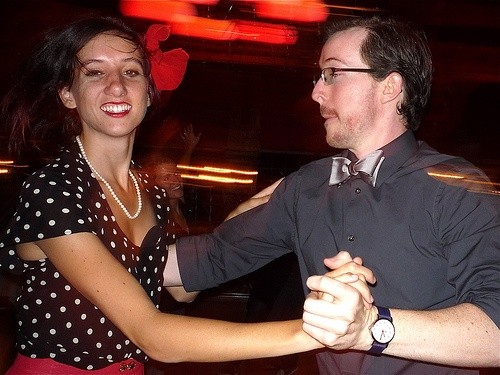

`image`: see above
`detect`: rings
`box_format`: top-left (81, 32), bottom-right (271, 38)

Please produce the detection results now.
top-left (183, 132), bottom-right (187, 136)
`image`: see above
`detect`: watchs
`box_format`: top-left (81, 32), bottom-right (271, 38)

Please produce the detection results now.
top-left (365, 304), bottom-right (396, 358)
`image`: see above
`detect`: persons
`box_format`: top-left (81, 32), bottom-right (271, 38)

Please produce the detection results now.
top-left (143, 123), bottom-right (204, 238)
top-left (163, 14), bottom-right (499, 375)
top-left (0, 15), bottom-right (379, 375)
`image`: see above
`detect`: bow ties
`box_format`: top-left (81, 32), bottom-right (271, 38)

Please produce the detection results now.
top-left (328, 150), bottom-right (385, 188)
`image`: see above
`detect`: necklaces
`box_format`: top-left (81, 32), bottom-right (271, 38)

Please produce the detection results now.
top-left (75, 135), bottom-right (142, 220)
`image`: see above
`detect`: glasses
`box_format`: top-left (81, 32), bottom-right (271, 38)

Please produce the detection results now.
top-left (313, 65), bottom-right (375, 86)
